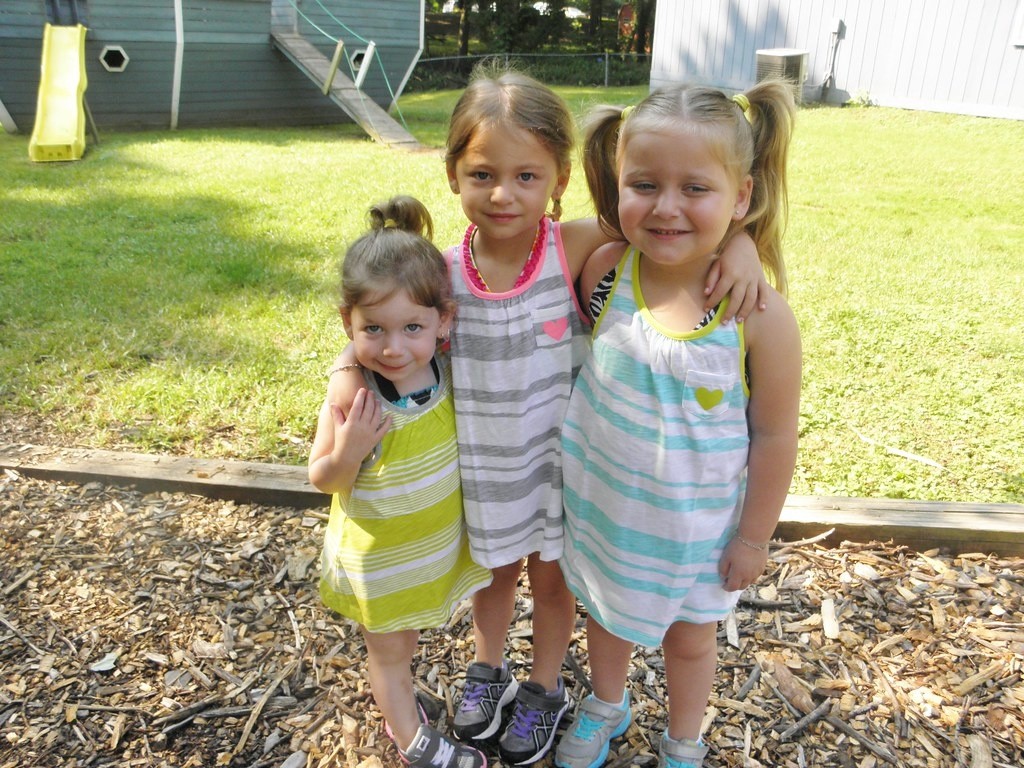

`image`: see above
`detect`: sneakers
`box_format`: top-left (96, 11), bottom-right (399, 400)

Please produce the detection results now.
top-left (658, 727), bottom-right (709, 768)
top-left (396, 722), bottom-right (486, 768)
top-left (385, 693), bottom-right (428, 743)
top-left (498, 671), bottom-right (568, 765)
top-left (555, 687), bottom-right (631, 768)
top-left (452, 657), bottom-right (518, 740)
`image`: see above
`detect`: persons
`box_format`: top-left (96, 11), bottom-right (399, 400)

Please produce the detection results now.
top-left (327, 72), bottom-right (770, 768)
top-left (308, 195), bottom-right (494, 768)
top-left (556, 78), bottom-right (803, 768)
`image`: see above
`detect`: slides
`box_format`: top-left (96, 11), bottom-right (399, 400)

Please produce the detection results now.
top-left (27, 23), bottom-right (88, 163)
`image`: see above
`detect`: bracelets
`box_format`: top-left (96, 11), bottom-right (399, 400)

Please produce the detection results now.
top-left (736, 532), bottom-right (767, 552)
top-left (328, 363), bottom-right (363, 377)
